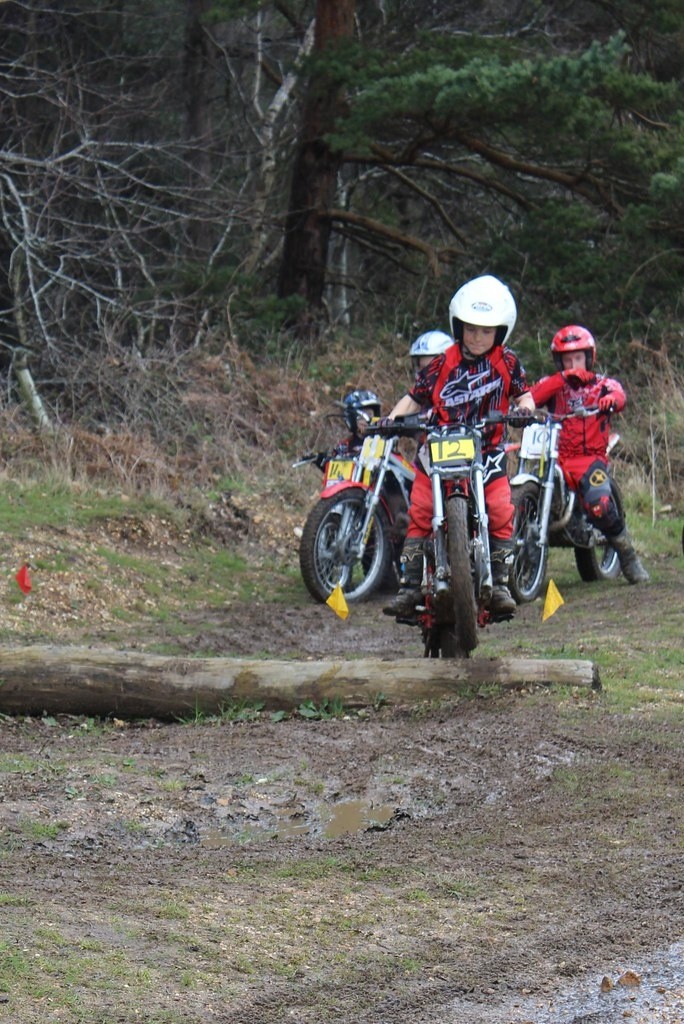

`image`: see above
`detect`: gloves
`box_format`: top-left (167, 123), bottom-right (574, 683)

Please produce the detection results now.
top-left (564, 368), bottom-right (595, 383)
top-left (370, 417), bottom-right (395, 439)
top-left (599, 395), bottom-right (616, 412)
top-left (299, 451), bottom-right (315, 463)
top-left (508, 406), bottom-right (531, 428)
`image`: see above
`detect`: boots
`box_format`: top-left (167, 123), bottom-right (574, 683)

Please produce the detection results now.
top-left (606, 526), bottom-right (648, 584)
top-left (382, 537), bottom-right (424, 616)
top-left (485, 535), bottom-right (516, 612)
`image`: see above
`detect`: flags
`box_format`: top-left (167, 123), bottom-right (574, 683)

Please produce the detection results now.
top-left (541, 579), bottom-right (564, 622)
top-left (15, 565), bottom-right (32, 594)
top-left (326, 583), bottom-right (348, 620)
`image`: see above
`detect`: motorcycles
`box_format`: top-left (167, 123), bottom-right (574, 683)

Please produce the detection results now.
top-left (507, 400), bottom-right (627, 605)
top-left (293, 450), bottom-right (360, 592)
top-left (300, 399), bottom-right (526, 604)
top-left (364, 410), bottom-right (547, 659)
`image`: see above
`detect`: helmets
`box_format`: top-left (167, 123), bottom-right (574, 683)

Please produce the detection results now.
top-left (343, 390), bottom-right (381, 432)
top-left (550, 325), bottom-right (596, 371)
top-left (449, 276), bottom-right (517, 346)
top-left (409, 331), bottom-right (456, 371)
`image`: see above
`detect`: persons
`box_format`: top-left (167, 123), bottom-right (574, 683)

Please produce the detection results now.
top-left (528, 324), bottom-right (652, 587)
top-left (303, 326), bottom-right (457, 598)
top-left (364, 274), bottom-right (539, 626)
